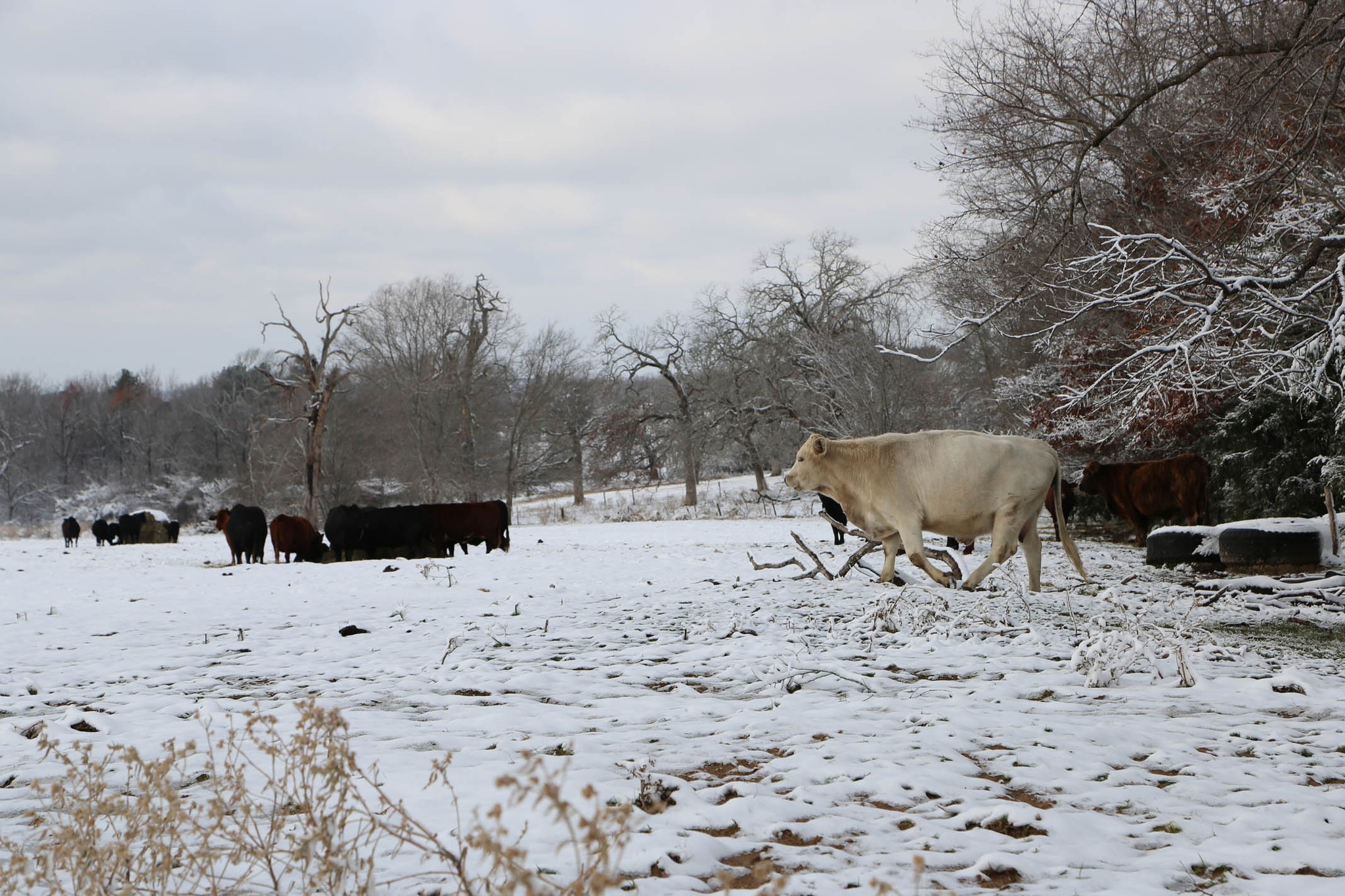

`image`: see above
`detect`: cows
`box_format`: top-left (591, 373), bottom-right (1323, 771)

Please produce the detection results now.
top-left (783, 433), bottom-right (1212, 594)
top-left (208, 500), bottom-right (510, 563)
top-left (62, 512), bottom-right (180, 547)
